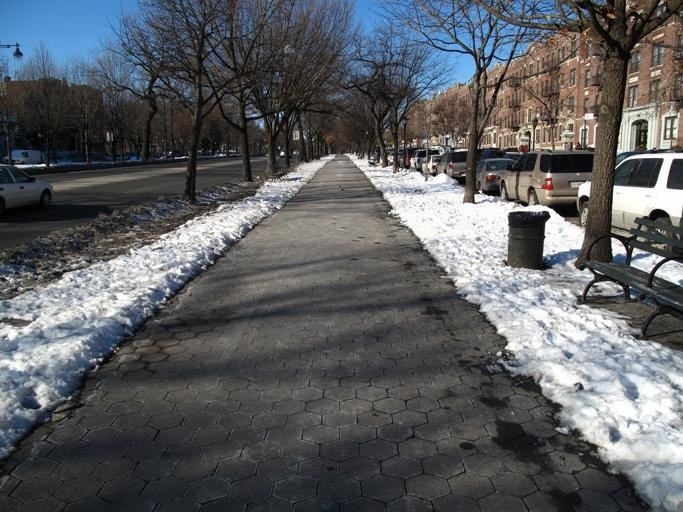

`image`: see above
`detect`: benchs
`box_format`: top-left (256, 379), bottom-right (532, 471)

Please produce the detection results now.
top-left (578, 217), bottom-right (682, 338)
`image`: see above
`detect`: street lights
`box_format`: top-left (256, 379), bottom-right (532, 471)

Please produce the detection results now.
top-left (402, 115), bottom-right (408, 168)
top-left (530, 117), bottom-right (540, 153)
top-left (1, 42), bottom-right (24, 62)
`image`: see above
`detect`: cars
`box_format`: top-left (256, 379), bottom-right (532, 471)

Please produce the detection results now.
top-left (0, 165), bottom-right (52, 212)
top-left (164, 151), bottom-right (177, 157)
top-left (264, 142), bottom-right (293, 158)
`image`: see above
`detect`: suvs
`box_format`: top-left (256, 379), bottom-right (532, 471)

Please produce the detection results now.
top-left (575, 153), bottom-right (683, 248)
top-left (378, 145), bottom-right (653, 209)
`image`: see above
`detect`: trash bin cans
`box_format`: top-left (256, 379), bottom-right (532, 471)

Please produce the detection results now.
top-left (507, 211), bottom-right (549, 269)
